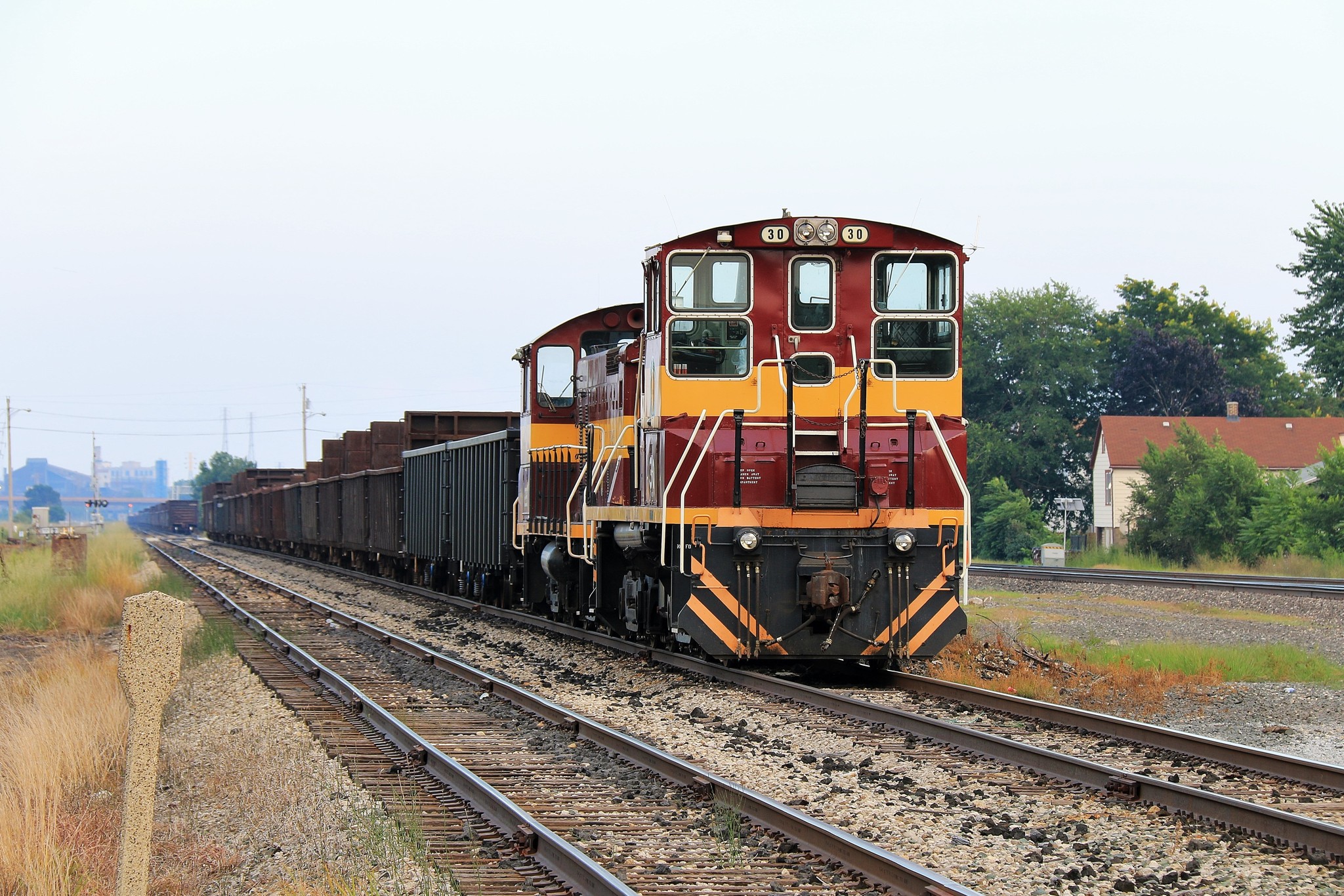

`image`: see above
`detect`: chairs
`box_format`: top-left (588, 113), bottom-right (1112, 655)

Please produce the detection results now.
top-left (887, 321), bottom-right (940, 374)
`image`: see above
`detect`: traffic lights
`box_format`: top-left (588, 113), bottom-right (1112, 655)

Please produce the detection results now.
top-left (84, 500), bottom-right (97, 508)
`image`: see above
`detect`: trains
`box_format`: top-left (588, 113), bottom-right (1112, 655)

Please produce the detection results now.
top-left (135, 499), bottom-right (199, 536)
top-left (199, 206), bottom-right (975, 679)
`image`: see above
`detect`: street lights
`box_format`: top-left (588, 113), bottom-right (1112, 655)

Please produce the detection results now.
top-left (6, 395), bottom-right (32, 539)
